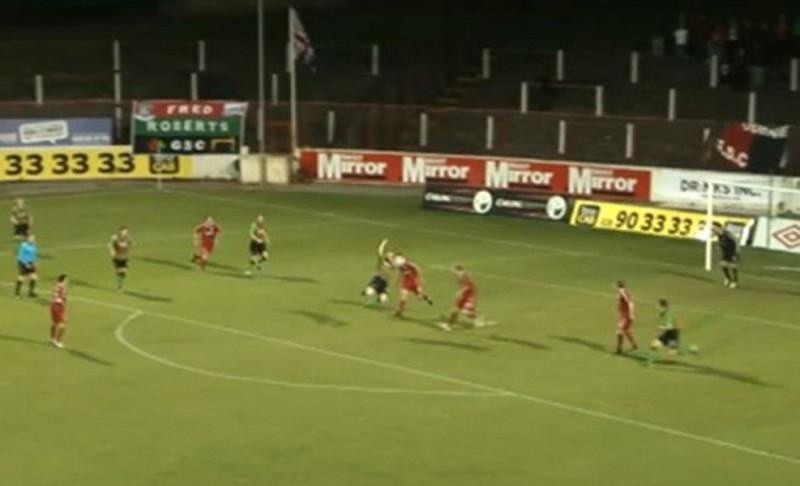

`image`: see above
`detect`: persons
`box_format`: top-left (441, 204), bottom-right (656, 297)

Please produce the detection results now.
top-left (188, 214), bottom-right (222, 273)
top-left (435, 263), bottom-right (495, 331)
top-left (380, 248), bottom-right (434, 318)
top-left (10, 197), bottom-right (34, 253)
top-left (13, 233), bottom-right (39, 302)
top-left (708, 221), bottom-right (741, 292)
top-left (611, 278), bottom-right (638, 357)
top-left (108, 224), bottom-right (135, 290)
top-left (650, 297), bottom-right (685, 358)
top-left (244, 213), bottom-right (273, 277)
top-left (50, 272), bottom-right (70, 350)
top-left (359, 237), bottom-right (397, 307)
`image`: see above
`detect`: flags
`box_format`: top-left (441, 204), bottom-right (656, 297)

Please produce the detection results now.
top-left (293, 9), bottom-right (317, 71)
